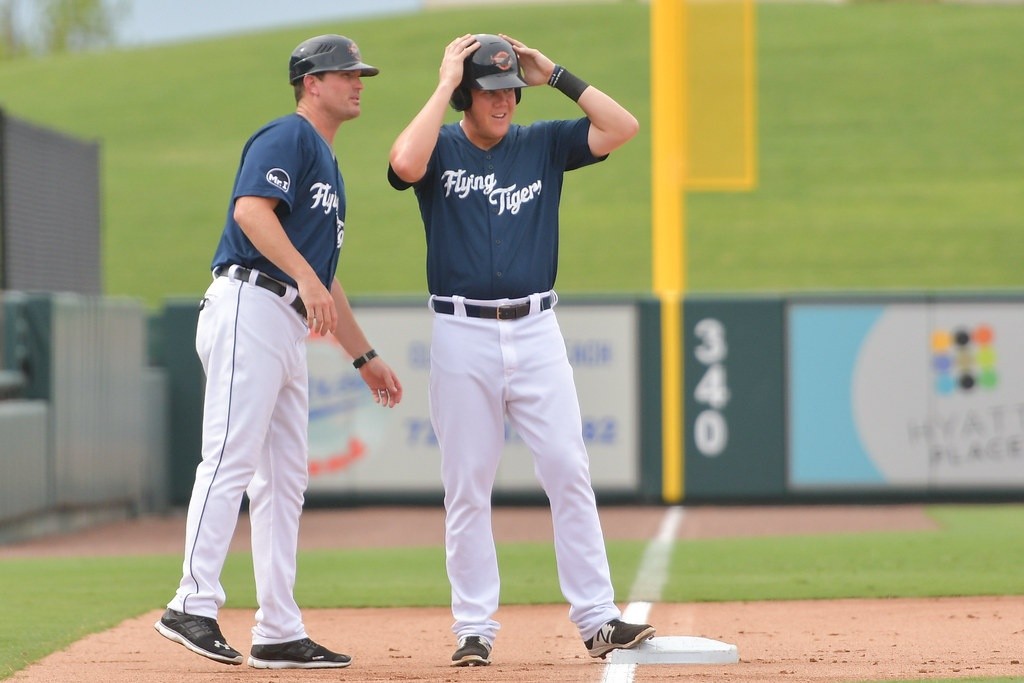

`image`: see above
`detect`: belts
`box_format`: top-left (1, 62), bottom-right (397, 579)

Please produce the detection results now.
top-left (434, 296), bottom-right (552, 321)
top-left (220, 266), bottom-right (307, 318)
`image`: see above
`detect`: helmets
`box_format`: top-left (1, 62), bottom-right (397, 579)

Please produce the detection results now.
top-left (447, 34), bottom-right (529, 112)
top-left (289, 34), bottom-right (379, 85)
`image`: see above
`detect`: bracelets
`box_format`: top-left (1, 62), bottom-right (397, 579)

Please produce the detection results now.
top-left (548, 65), bottom-right (591, 103)
top-left (352, 349), bottom-right (377, 369)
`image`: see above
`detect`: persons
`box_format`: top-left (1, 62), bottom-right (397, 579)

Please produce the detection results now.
top-left (387, 32), bottom-right (654, 667)
top-left (152, 33), bottom-right (402, 670)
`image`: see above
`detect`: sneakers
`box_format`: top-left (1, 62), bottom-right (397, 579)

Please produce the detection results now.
top-left (247, 636), bottom-right (351, 669)
top-left (451, 635), bottom-right (491, 667)
top-left (585, 619), bottom-right (656, 660)
top-left (153, 609), bottom-right (244, 666)
